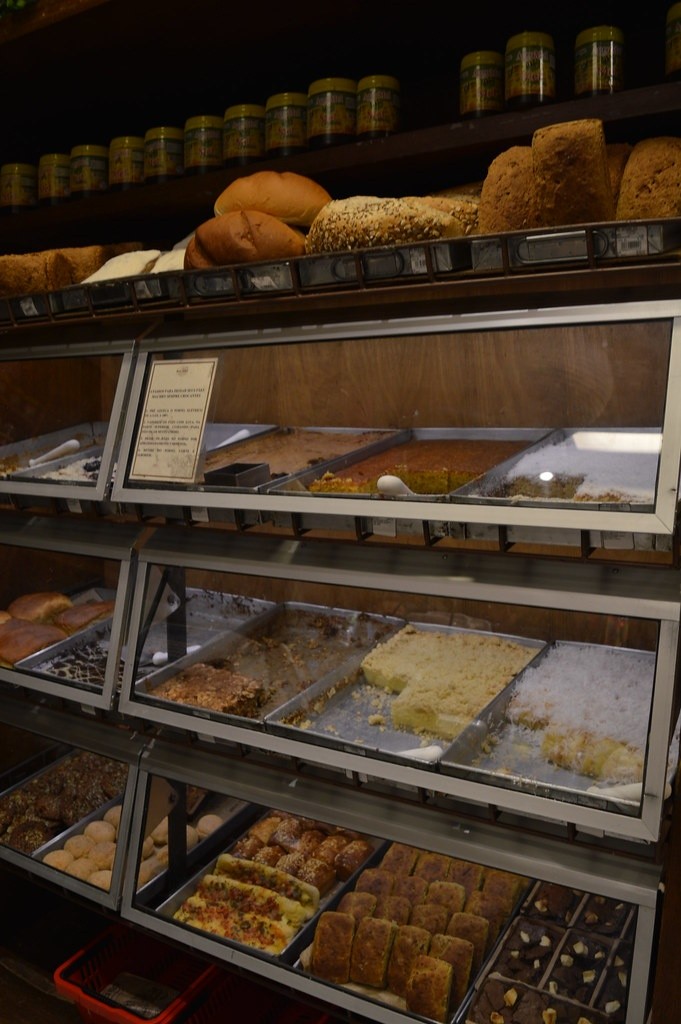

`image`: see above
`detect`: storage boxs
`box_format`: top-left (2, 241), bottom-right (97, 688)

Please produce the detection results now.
top-left (54, 920), bottom-right (221, 1024)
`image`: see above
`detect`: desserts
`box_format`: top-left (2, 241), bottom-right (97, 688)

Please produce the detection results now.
top-left (134, 429), bottom-right (668, 801)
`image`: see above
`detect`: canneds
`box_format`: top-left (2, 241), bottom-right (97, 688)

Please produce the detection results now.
top-left (1, 25), bottom-right (623, 210)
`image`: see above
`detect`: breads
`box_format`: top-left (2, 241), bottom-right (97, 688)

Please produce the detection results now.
top-left (0, 750), bottom-right (634, 1024)
top-left (0, 590), bottom-right (115, 668)
top-left (0, 116), bottom-right (681, 297)
